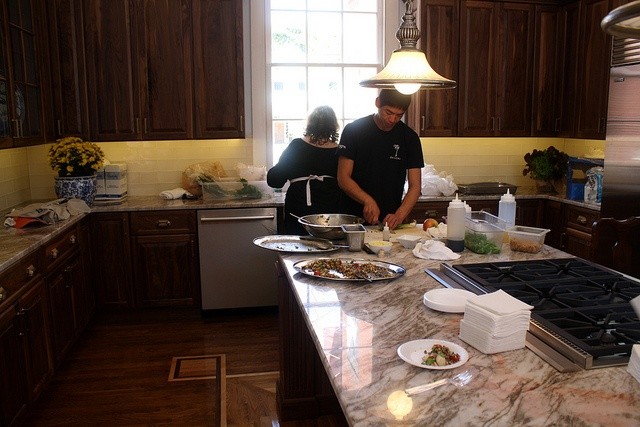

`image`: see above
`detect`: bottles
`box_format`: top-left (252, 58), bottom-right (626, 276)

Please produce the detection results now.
top-left (447, 192), bottom-right (466, 252)
top-left (498, 188), bottom-right (516, 233)
top-left (383, 222), bottom-right (390, 242)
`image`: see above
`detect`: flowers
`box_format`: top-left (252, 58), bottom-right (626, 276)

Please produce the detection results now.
top-left (46, 136), bottom-right (104, 177)
top-left (523, 145), bottom-right (557, 178)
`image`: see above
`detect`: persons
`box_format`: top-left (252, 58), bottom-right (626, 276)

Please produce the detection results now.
top-left (267, 106), bottom-right (339, 235)
top-left (337, 89), bottom-right (424, 231)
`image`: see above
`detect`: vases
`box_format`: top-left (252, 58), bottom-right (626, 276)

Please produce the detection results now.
top-left (54, 177), bottom-right (98, 208)
top-left (535, 178), bottom-right (554, 191)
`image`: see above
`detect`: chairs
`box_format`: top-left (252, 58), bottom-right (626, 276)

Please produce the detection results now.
top-left (593, 217), bottom-right (640, 280)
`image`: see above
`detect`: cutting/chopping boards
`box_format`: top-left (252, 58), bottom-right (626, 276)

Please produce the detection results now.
top-left (362, 223), bottom-right (431, 242)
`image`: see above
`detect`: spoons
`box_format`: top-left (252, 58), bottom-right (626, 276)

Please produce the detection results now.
top-left (314, 241), bottom-right (350, 249)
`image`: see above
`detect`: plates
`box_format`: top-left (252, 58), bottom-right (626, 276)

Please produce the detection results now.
top-left (253, 235), bottom-right (341, 252)
top-left (397, 339), bottom-right (469, 370)
top-left (423, 289), bottom-right (477, 314)
top-left (293, 258), bottom-right (406, 281)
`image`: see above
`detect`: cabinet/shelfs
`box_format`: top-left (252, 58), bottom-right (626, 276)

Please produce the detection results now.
top-left (130, 210), bottom-right (204, 322)
top-left (404, 202), bottom-right (450, 226)
top-left (0, 212), bottom-right (99, 427)
top-left (459, 0), bottom-right (534, 136)
top-left (48, 0), bottom-right (245, 143)
top-left (406, 0), bottom-right (460, 135)
top-left (562, 203), bottom-right (600, 264)
top-left (89, 211), bottom-right (132, 321)
top-left (467, 200), bottom-right (545, 228)
top-left (532, 1), bottom-right (613, 140)
top-left (539, 199), bottom-right (561, 239)
top-left (0, 0), bottom-right (51, 149)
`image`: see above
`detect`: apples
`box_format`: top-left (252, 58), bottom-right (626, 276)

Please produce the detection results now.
top-left (423, 218), bottom-right (438, 231)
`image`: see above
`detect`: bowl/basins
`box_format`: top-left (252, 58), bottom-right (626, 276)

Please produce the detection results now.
top-left (298, 214), bottom-right (365, 239)
top-left (397, 234), bottom-right (423, 248)
top-left (369, 241), bottom-right (393, 253)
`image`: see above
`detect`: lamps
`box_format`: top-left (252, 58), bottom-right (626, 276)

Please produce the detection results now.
top-left (360, 0), bottom-right (457, 95)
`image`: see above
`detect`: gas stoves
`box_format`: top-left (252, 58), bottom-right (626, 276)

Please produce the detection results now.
top-left (425, 257), bottom-right (640, 373)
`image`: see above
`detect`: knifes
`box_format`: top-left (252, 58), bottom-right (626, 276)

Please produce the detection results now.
top-left (378, 220), bottom-right (385, 231)
